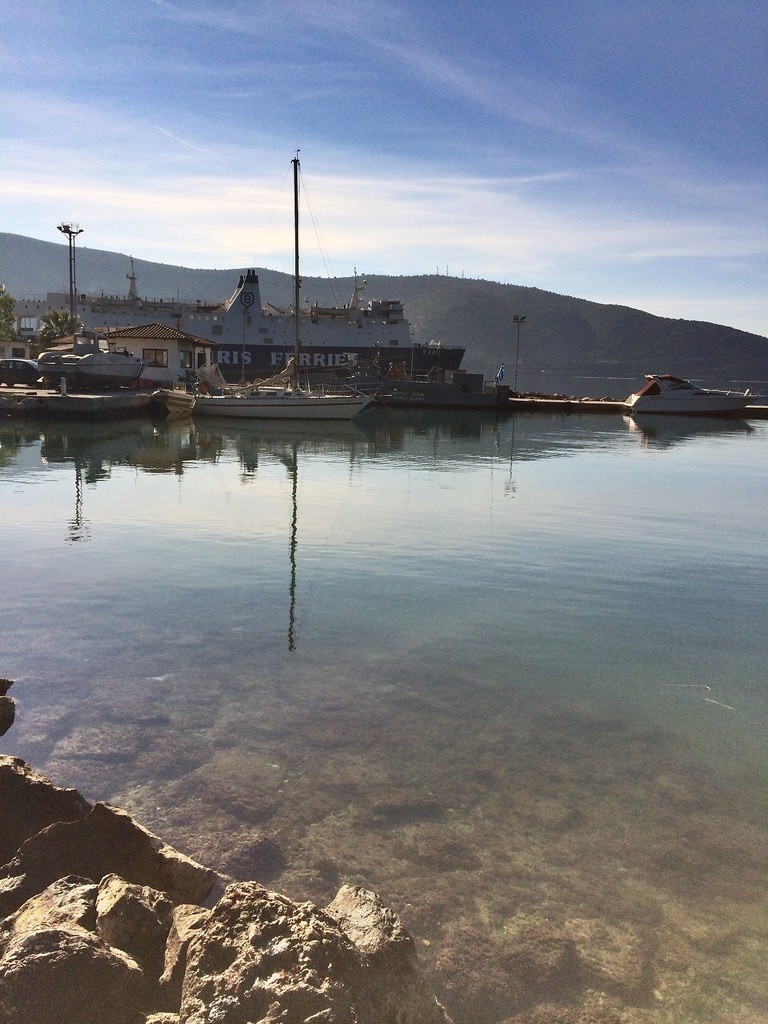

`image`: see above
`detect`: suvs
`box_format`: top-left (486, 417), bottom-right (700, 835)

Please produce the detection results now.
top-left (1, 359), bottom-right (40, 388)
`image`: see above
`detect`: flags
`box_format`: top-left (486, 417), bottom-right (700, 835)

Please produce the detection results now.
top-left (496, 365), bottom-right (504, 382)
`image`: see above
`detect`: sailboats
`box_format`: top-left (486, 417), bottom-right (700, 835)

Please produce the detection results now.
top-left (190, 413), bottom-right (367, 651)
top-left (188, 148), bottom-right (384, 421)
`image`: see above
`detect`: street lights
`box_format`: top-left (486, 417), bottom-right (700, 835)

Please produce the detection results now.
top-left (512, 314), bottom-right (529, 392)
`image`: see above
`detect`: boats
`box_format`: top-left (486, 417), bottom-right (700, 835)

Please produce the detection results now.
top-left (37, 415), bottom-right (156, 483)
top-left (151, 379), bottom-right (194, 413)
top-left (622, 373), bottom-right (760, 413)
top-left (37, 330), bottom-right (150, 388)
